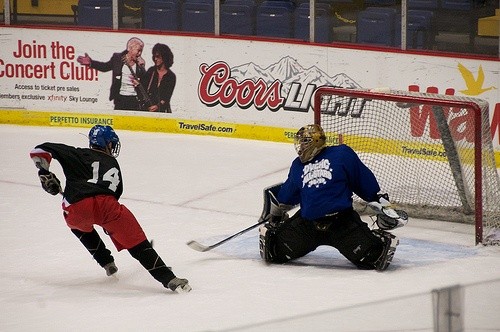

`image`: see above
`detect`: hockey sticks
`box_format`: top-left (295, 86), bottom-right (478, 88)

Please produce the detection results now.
top-left (186, 203), bottom-right (300, 252)
top-left (60, 190), bottom-right (153, 247)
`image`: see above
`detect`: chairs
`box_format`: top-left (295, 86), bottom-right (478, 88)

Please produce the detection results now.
top-left (71, 0), bottom-right (498, 49)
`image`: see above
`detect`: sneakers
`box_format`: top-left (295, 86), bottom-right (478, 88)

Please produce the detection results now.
top-left (167, 276), bottom-right (192, 295)
top-left (104, 262), bottom-right (119, 280)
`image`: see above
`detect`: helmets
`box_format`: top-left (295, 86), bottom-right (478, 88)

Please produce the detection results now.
top-left (293, 124), bottom-right (326, 163)
top-left (88, 124), bottom-right (121, 158)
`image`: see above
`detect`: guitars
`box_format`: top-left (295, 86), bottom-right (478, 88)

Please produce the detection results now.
top-left (120, 54), bottom-right (156, 111)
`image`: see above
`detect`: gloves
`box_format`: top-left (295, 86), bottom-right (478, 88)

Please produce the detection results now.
top-left (38, 169), bottom-right (61, 195)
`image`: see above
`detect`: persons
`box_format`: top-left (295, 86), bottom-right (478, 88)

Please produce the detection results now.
top-left (258, 124), bottom-right (408, 271)
top-left (30, 124), bottom-right (189, 291)
top-left (133, 44), bottom-right (176, 113)
top-left (78, 37), bottom-right (145, 111)
